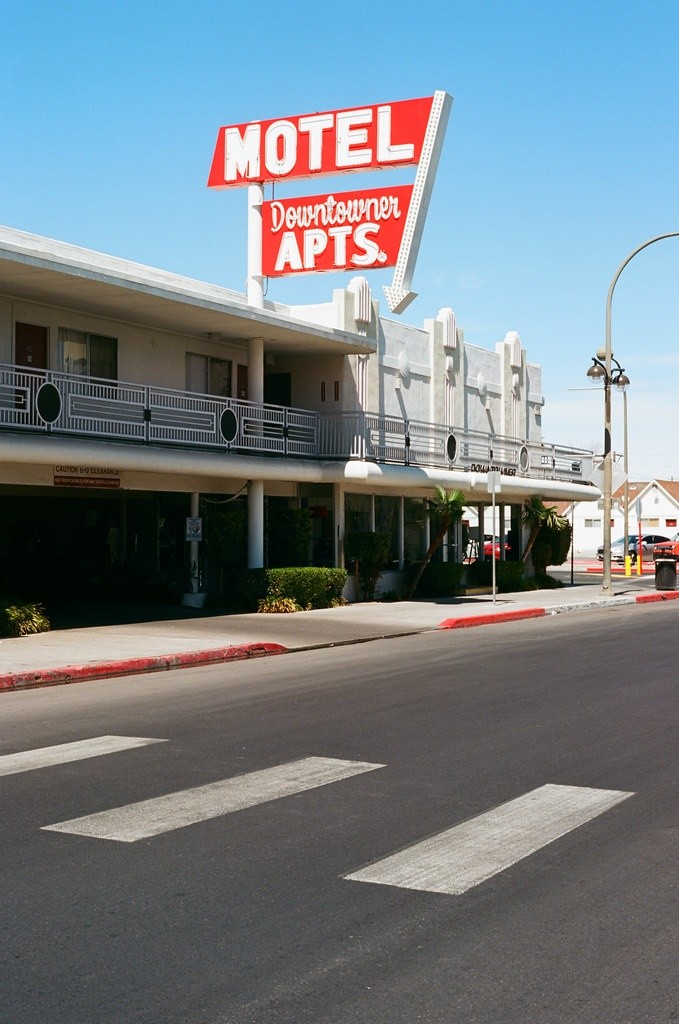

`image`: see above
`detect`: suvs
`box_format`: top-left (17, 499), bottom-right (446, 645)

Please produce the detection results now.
top-left (653, 533), bottom-right (679, 565)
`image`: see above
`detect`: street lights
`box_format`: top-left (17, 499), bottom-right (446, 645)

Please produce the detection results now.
top-left (587, 358), bottom-right (629, 594)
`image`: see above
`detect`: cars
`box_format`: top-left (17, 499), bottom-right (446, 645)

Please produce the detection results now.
top-left (484, 534), bottom-right (511, 562)
top-left (596, 533), bottom-right (671, 565)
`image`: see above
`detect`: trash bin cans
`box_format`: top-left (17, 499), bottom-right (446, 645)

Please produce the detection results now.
top-left (653, 553), bottom-right (679, 590)
